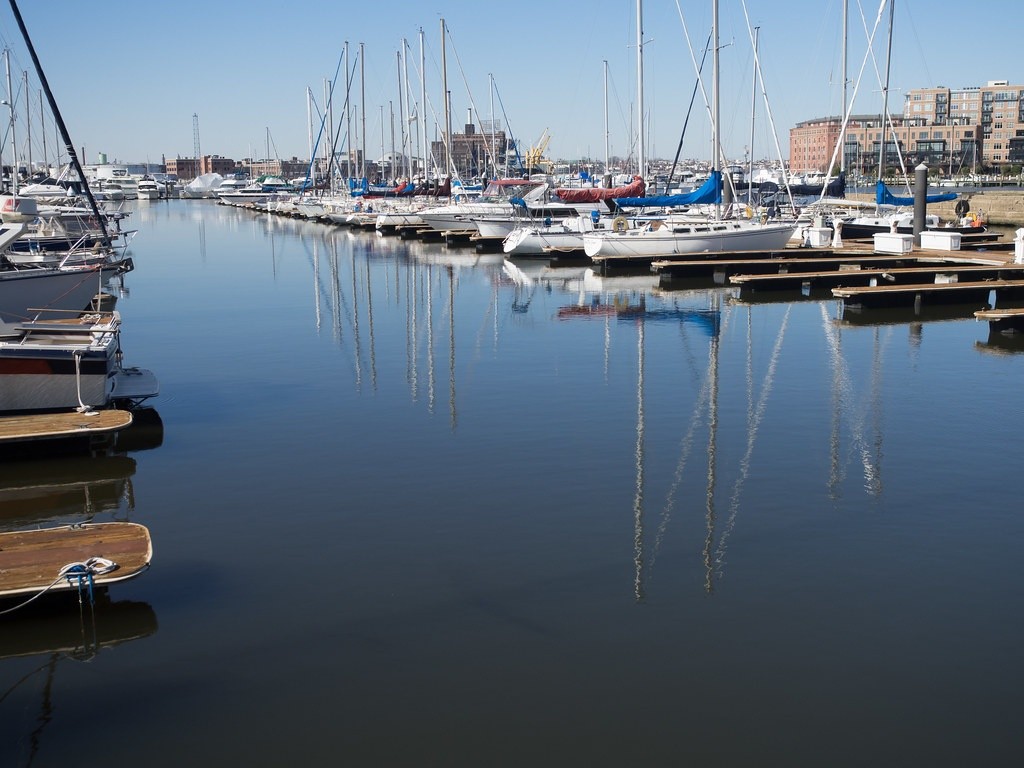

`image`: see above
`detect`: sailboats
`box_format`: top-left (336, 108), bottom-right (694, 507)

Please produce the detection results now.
top-left (0, 1), bottom-right (160, 411)
top-left (222, 0), bottom-right (873, 259)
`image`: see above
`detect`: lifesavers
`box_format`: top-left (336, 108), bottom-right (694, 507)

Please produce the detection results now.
top-left (956, 200), bottom-right (969, 218)
top-left (614, 295), bottom-right (629, 313)
top-left (966, 212), bottom-right (977, 222)
top-left (746, 207), bottom-right (752, 219)
top-left (612, 217), bottom-right (629, 232)
